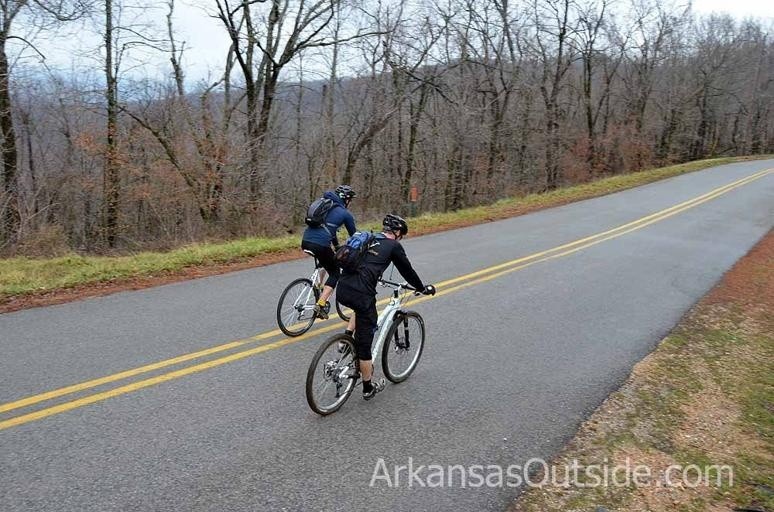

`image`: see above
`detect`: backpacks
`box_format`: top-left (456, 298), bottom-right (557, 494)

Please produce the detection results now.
top-left (333, 230), bottom-right (386, 272)
top-left (305, 198), bottom-right (340, 238)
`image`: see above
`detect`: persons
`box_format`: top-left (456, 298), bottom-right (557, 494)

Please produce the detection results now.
top-left (336, 214), bottom-right (435, 400)
top-left (301, 184), bottom-right (356, 319)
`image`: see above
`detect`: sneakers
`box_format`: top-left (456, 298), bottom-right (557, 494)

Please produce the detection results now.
top-left (362, 378), bottom-right (386, 401)
top-left (337, 341), bottom-right (351, 353)
top-left (312, 303), bottom-right (329, 319)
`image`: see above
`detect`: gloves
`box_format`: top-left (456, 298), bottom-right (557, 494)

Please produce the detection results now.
top-left (425, 284), bottom-right (436, 296)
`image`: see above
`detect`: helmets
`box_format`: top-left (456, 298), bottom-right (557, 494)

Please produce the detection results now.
top-left (381, 214), bottom-right (408, 235)
top-left (335, 185), bottom-right (356, 204)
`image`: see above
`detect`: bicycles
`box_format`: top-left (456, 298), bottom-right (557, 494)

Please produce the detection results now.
top-left (277, 244), bottom-right (354, 337)
top-left (306, 275), bottom-right (425, 415)
